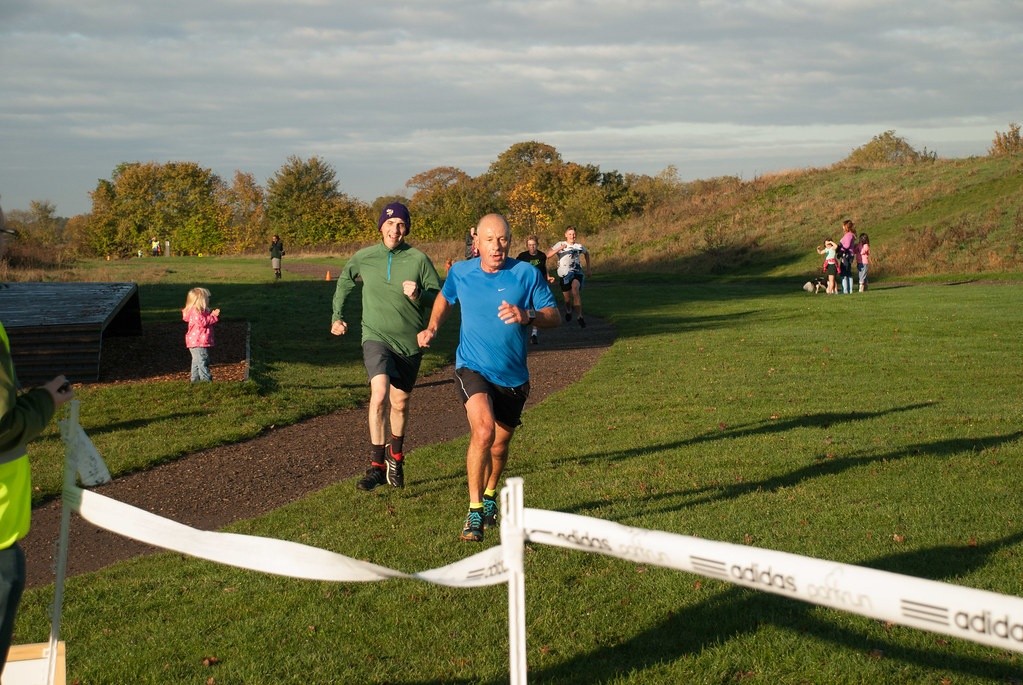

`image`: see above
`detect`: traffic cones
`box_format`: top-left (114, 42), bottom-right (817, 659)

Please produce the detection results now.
top-left (325, 270), bottom-right (331, 281)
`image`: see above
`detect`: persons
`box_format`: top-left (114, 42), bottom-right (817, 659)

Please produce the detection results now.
top-left (547, 226), bottom-right (591, 328)
top-left (465, 226), bottom-right (477, 259)
top-left (817, 236), bottom-right (838, 294)
top-left (516, 234), bottom-right (556, 344)
top-left (330, 204), bottom-right (441, 491)
top-left (854, 233), bottom-right (871, 292)
top-left (417, 214), bottom-right (561, 540)
top-left (836, 220), bottom-right (858, 294)
top-left (182, 288), bottom-right (220, 382)
top-left (270, 235), bottom-right (283, 280)
top-left (0, 207), bottom-right (75, 675)
top-left (149, 237), bottom-right (160, 256)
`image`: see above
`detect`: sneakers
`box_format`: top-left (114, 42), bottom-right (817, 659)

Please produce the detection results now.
top-left (565, 307), bottom-right (572, 322)
top-left (461, 505), bottom-right (486, 541)
top-left (481, 490), bottom-right (499, 529)
top-left (577, 316), bottom-right (586, 328)
top-left (384, 443), bottom-right (403, 489)
top-left (530, 334), bottom-right (537, 345)
top-left (358, 464), bottom-right (387, 489)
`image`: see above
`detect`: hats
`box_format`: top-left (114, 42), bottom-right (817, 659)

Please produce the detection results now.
top-left (377, 203), bottom-right (410, 236)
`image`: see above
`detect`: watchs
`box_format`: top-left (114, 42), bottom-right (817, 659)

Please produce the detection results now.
top-left (525, 306), bottom-right (536, 325)
